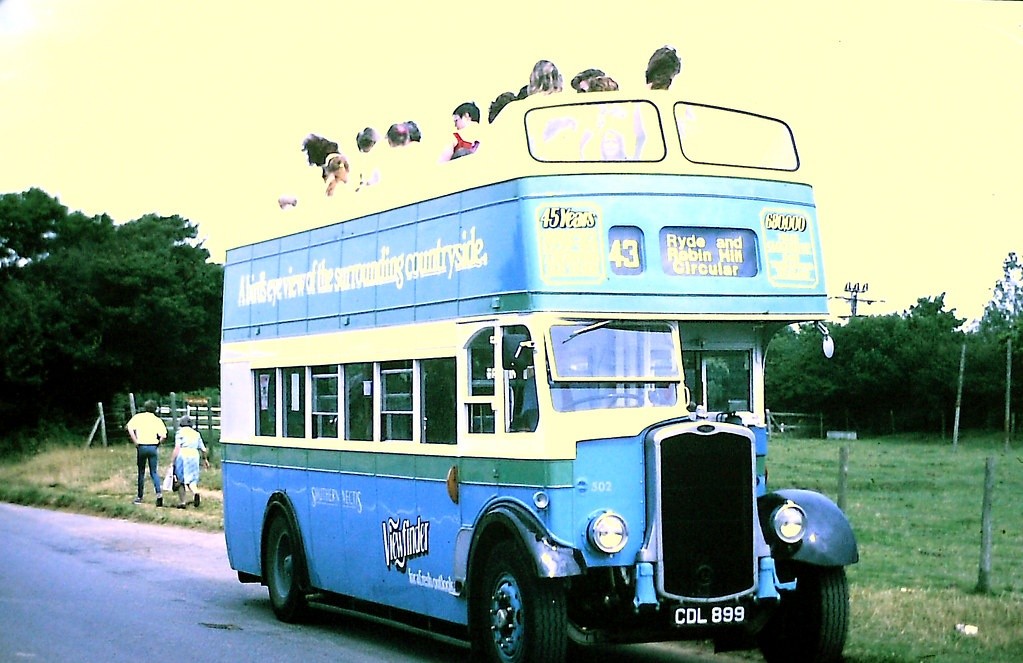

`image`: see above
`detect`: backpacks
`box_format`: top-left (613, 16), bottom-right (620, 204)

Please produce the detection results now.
top-left (452, 132), bottom-right (472, 152)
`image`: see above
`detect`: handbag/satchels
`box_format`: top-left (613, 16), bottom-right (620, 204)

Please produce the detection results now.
top-left (172, 464), bottom-right (180, 492)
top-left (163, 465), bottom-right (174, 492)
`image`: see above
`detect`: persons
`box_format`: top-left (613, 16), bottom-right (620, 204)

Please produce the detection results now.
top-left (127, 400), bottom-right (168, 506)
top-left (299, 46), bottom-right (683, 194)
top-left (169, 414), bottom-right (210, 509)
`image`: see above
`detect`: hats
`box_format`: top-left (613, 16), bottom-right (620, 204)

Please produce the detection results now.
top-left (144, 400), bottom-right (158, 408)
top-left (180, 416), bottom-right (193, 427)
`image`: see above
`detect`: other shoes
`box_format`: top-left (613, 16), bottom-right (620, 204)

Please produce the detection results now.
top-left (195, 493), bottom-right (201, 507)
top-left (177, 504), bottom-right (187, 509)
top-left (132, 497), bottom-right (143, 505)
top-left (156, 497), bottom-right (163, 507)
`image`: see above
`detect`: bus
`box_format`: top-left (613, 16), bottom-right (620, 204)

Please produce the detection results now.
top-left (217, 91), bottom-right (858, 662)
